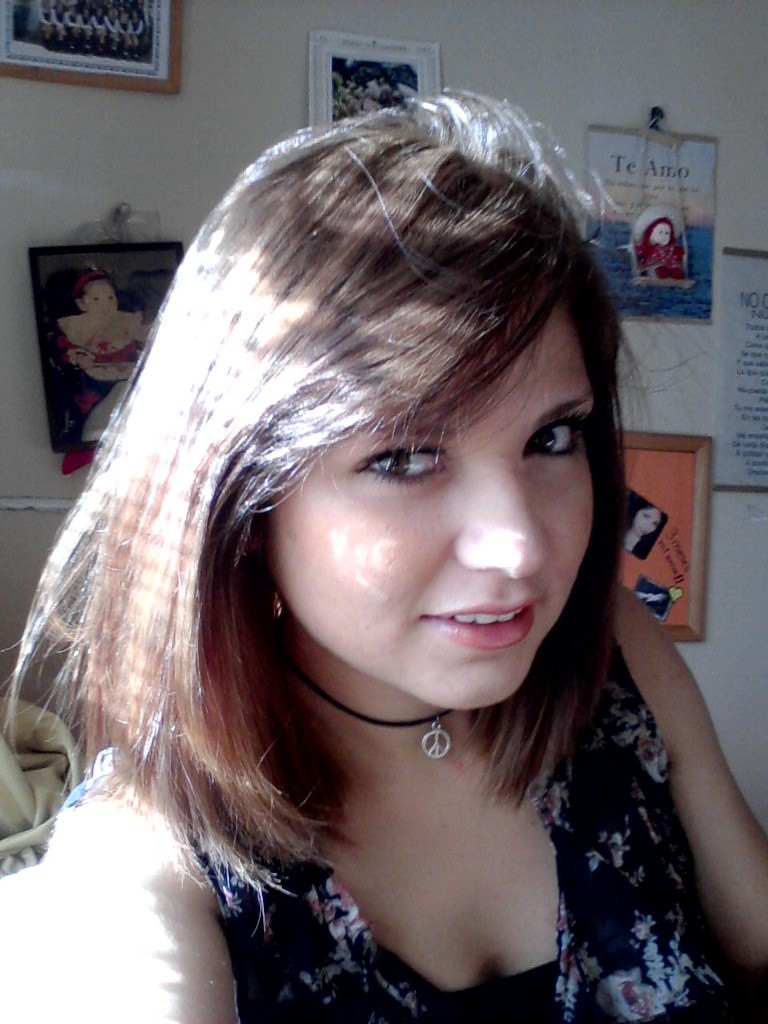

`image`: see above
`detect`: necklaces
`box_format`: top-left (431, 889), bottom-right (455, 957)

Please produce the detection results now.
top-left (282, 652), bottom-right (453, 759)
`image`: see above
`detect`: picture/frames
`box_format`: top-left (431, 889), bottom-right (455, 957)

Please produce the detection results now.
top-left (27, 241), bottom-right (184, 454)
top-left (308, 29), bottom-right (441, 128)
top-left (611, 430), bottom-right (711, 644)
top-left (0, 1), bottom-right (183, 95)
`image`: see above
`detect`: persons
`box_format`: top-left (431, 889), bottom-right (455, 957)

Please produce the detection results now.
top-left (621, 503), bottom-right (662, 559)
top-left (360, 89), bottom-right (392, 115)
top-left (27, 0), bottom-right (149, 60)
top-left (637, 591), bottom-right (670, 613)
top-left (53, 268), bottom-right (146, 419)
top-left (633, 207), bottom-right (690, 280)
top-left (2, 89), bottom-right (768, 1024)
